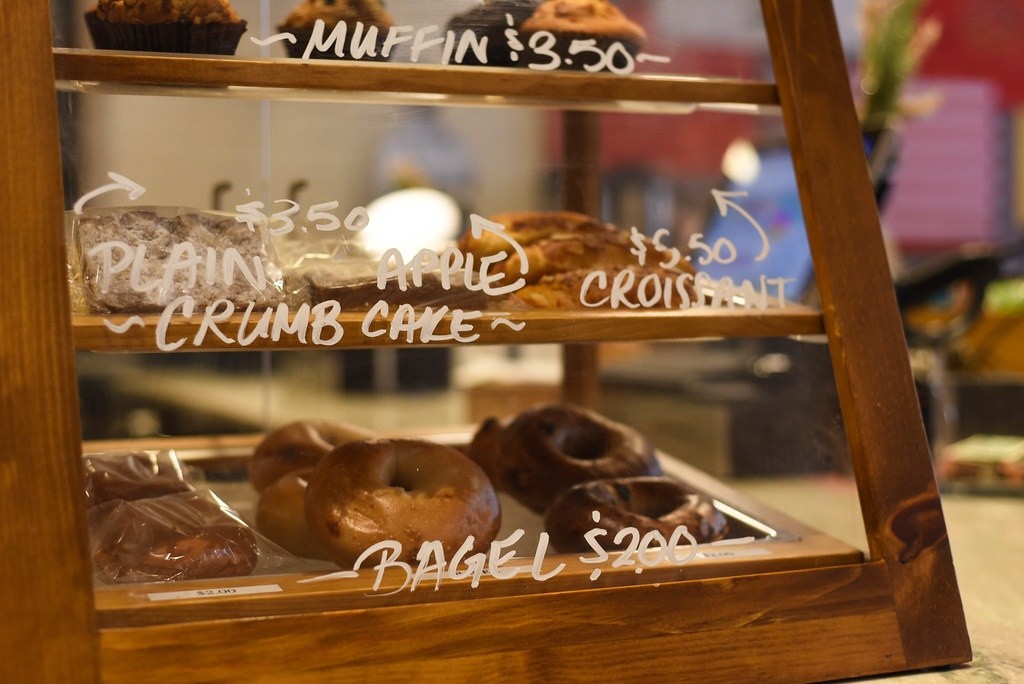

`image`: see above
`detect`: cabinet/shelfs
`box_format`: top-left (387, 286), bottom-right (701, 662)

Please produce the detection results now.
top-left (0, 0), bottom-right (974, 684)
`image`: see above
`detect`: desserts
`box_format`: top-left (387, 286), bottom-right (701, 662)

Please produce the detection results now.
top-left (82, 0), bottom-right (649, 75)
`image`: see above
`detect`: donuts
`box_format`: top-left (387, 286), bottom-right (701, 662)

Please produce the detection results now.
top-left (82, 402), bottom-right (732, 588)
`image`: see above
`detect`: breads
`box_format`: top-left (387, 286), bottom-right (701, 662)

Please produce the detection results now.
top-left (63, 206), bottom-right (703, 319)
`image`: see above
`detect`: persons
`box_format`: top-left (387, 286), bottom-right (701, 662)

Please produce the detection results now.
top-left (333, 96), bottom-right (484, 397)
top-left (807, 1), bottom-right (1024, 493)
